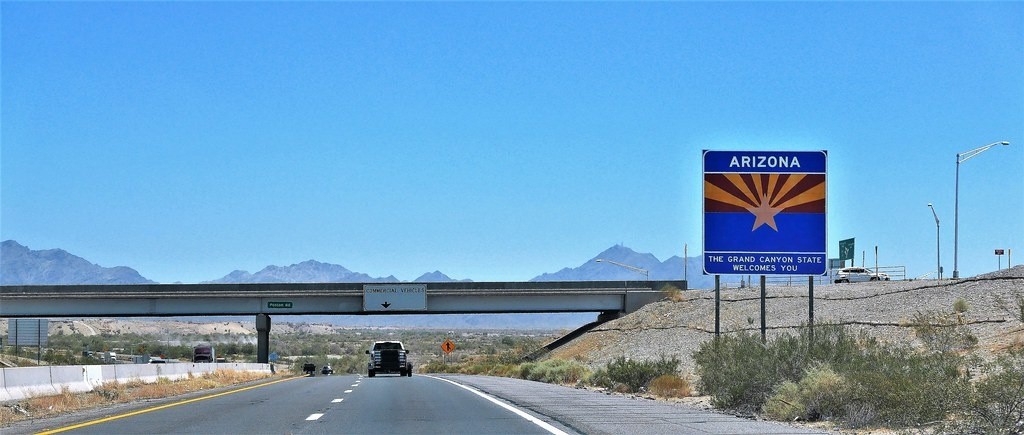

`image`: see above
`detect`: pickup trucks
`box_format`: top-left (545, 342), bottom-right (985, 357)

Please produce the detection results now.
top-left (365, 340), bottom-right (413, 377)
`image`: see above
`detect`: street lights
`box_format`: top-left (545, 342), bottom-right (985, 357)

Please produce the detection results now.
top-left (953, 142), bottom-right (1010, 278)
top-left (928, 203), bottom-right (940, 279)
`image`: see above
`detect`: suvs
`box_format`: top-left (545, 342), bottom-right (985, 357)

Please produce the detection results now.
top-left (834, 267), bottom-right (891, 283)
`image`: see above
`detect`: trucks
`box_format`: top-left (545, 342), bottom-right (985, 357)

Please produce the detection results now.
top-left (193, 345), bottom-right (215, 363)
